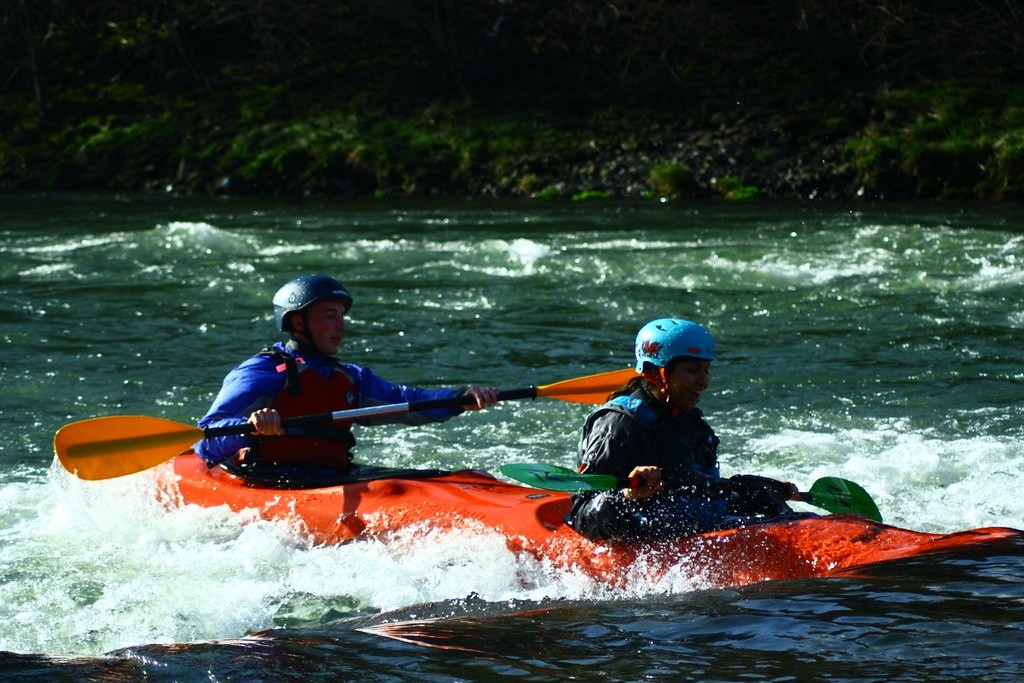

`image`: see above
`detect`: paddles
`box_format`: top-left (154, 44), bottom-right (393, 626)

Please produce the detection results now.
top-left (52, 367), bottom-right (648, 483)
top-left (498, 462), bottom-right (885, 525)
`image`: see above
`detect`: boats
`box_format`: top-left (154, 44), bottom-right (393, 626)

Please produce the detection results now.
top-left (52, 421), bottom-right (1023, 593)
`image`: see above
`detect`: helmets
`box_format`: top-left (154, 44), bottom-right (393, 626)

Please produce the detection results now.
top-left (633, 319), bottom-right (715, 375)
top-left (273, 274), bottom-right (354, 332)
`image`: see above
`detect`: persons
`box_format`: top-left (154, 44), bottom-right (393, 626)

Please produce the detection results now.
top-left (569, 318), bottom-right (800, 544)
top-left (191, 275), bottom-right (499, 480)
